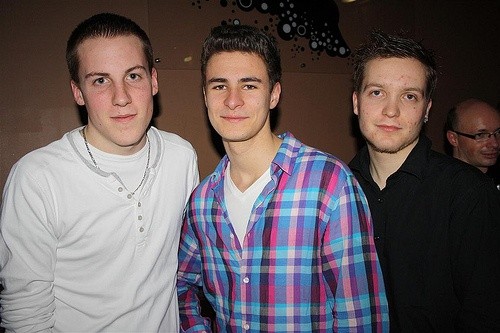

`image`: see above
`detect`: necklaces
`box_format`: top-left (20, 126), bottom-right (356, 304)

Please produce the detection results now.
top-left (82, 125), bottom-right (150, 194)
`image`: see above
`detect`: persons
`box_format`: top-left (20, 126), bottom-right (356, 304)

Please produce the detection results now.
top-left (0, 10), bottom-right (201, 333)
top-left (442, 98), bottom-right (500, 193)
top-left (347, 28), bottom-right (500, 333)
top-left (176, 22), bottom-right (390, 333)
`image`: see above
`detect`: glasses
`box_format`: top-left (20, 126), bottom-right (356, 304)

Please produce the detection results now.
top-left (455, 128), bottom-right (500, 142)
